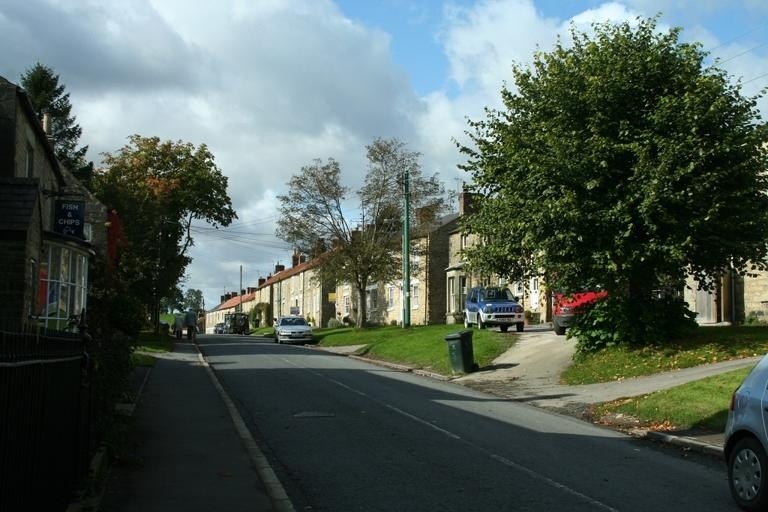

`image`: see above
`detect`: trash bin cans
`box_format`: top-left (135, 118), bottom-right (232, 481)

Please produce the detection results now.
top-left (445, 330), bottom-right (479, 376)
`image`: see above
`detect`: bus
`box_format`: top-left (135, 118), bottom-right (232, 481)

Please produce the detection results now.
top-left (224, 312), bottom-right (250, 336)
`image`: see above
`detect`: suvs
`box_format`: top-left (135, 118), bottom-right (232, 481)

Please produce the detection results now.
top-left (463, 285), bottom-right (524, 334)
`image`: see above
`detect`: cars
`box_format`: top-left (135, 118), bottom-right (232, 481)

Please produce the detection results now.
top-left (272, 316), bottom-right (313, 346)
top-left (146, 318), bottom-right (171, 331)
top-left (551, 286), bottom-right (611, 336)
top-left (722, 352), bottom-right (767, 512)
top-left (212, 322), bottom-right (226, 335)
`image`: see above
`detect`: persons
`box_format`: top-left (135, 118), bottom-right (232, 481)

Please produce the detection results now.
top-left (185, 308), bottom-right (197, 343)
top-left (173, 316), bottom-right (184, 343)
